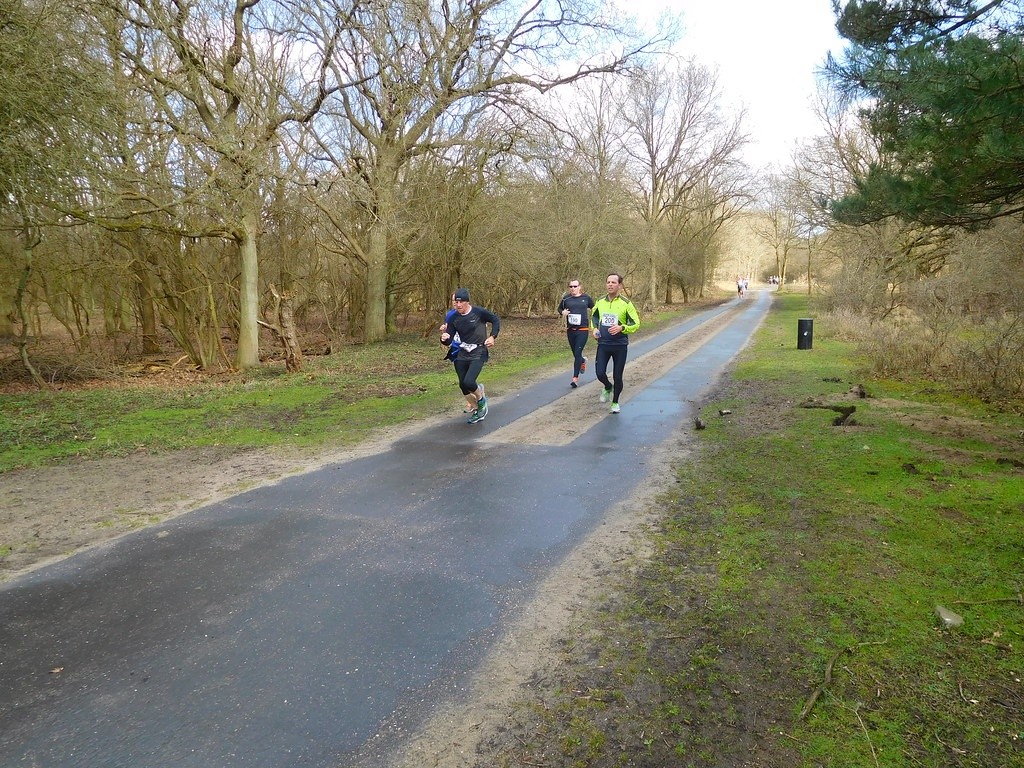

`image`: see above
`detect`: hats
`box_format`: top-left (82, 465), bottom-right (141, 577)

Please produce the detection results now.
top-left (455, 288), bottom-right (469, 301)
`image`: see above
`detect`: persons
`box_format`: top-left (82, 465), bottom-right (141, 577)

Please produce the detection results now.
top-left (769, 275), bottom-right (779, 286)
top-left (558, 280), bottom-right (595, 388)
top-left (736, 274), bottom-right (748, 297)
top-left (591, 274), bottom-right (640, 413)
top-left (440, 290), bottom-right (500, 423)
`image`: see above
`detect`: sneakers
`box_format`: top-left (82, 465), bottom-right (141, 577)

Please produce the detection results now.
top-left (467, 413), bottom-right (479, 423)
top-left (464, 405), bottom-right (474, 413)
top-left (611, 402), bottom-right (620, 413)
top-left (600, 385), bottom-right (614, 401)
top-left (478, 383), bottom-right (484, 394)
top-left (570, 377), bottom-right (578, 388)
top-left (580, 357), bottom-right (587, 373)
top-left (477, 396), bottom-right (488, 420)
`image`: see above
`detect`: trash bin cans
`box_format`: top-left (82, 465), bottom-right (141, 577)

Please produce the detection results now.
top-left (797, 319), bottom-right (814, 349)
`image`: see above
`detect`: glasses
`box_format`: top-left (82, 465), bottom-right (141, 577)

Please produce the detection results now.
top-left (569, 285), bottom-right (579, 288)
